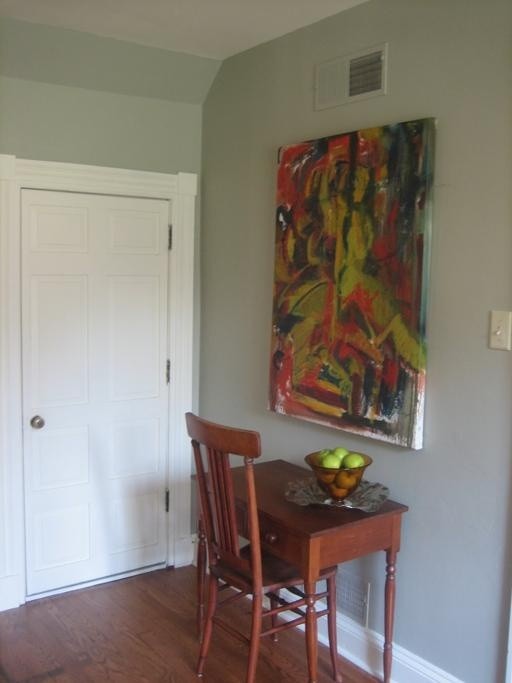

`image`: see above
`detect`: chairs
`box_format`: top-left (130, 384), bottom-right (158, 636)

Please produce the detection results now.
top-left (181, 412), bottom-right (335, 683)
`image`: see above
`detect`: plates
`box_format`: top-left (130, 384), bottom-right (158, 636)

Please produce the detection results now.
top-left (282, 475), bottom-right (391, 513)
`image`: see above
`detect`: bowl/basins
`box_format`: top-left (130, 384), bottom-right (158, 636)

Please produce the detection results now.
top-left (304, 448), bottom-right (375, 505)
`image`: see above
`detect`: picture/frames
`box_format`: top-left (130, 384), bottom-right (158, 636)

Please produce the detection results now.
top-left (264, 115), bottom-right (438, 451)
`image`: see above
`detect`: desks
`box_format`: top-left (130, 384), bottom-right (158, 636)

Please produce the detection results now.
top-left (191, 459), bottom-right (409, 683)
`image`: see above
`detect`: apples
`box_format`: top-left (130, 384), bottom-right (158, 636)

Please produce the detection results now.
top-left (337, 472), bottom-right (356, 489)
top-left (317, 449), bottom-right (330, 462)
top-left (331, 484), bottom-right (347, 498)
top-left (344, 453), bottom-right (365, 468)
top-left (322, 454), bottom-right (340, 469)
top-left (333, 448), bottom-right (349, 466)
top-left (319, 472), bottom-right (335, 483)
